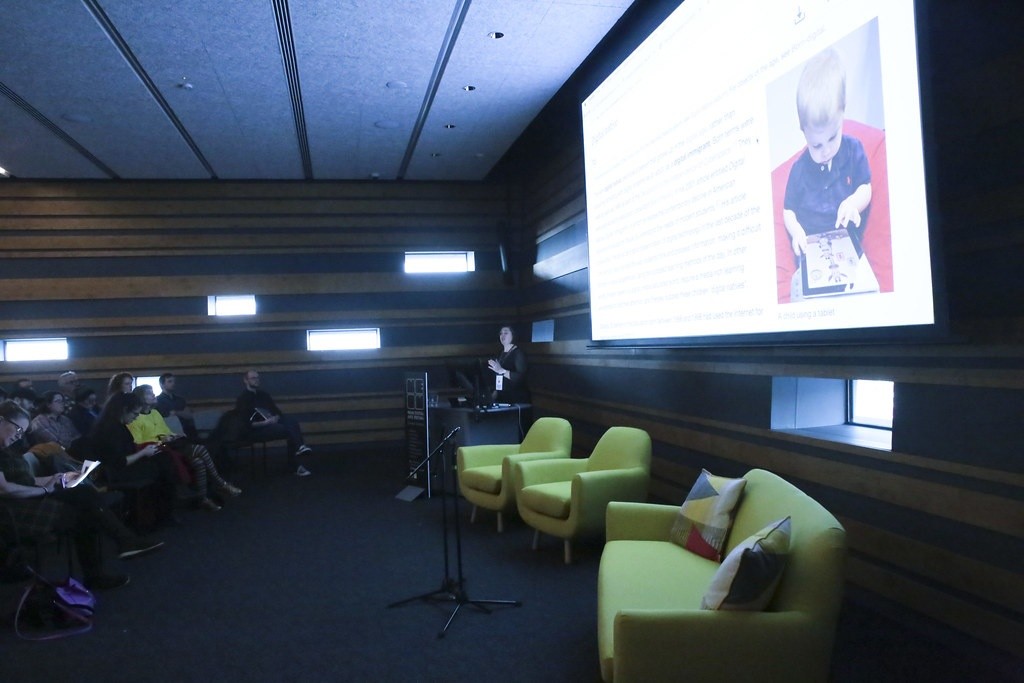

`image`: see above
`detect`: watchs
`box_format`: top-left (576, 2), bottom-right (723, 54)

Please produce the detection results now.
top-left (501, 369), bottom-right (508, 375)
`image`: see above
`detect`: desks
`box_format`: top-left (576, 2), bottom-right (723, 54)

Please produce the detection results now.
top-left (427, 399), bottom-right (534, 479)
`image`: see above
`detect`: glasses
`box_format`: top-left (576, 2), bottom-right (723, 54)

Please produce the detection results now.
top-left (4, 417), bottom-right (24, 439)
top-left (63, 378), bottom-right (81, 386)
top-left (247, 376), bottom-right (260, 381)
top-left (51, 399), bottom-right (64, 404)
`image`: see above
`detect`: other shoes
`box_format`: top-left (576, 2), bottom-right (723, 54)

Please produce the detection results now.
top-left (116, 534), bottom-right (165, 560)
top-left (291, 466), bottom-right (312, 477)
top-left (84, 572), bottom-right (131, 591)
top-left (198, 482), bottom-right (242, 512)
top-left (298, 445), bottom-right (312, 454)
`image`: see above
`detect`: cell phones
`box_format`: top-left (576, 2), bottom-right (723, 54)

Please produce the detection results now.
top-left (154, 442), bottom-right (166, 449)
top-left (61, 472), bottom-right (67, 488)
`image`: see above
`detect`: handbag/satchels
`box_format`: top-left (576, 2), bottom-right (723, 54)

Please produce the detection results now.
top-left (23, 569), bottom-right (97, 626)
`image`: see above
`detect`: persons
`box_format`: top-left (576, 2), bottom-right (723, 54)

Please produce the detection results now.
top-left (236, 370), bottom-right (314, 476)
top-left (487, 324), bottom-right (532, 405)
top-left (781, 53), bottom-right (875, 302)
top-left (0, 368), bottom-right (242, 594)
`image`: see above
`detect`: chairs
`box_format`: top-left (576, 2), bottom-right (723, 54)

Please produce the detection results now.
top-left (515, 424), bottom-right (652, 565)
top-left (217, 410), bottom-right (268, 474)
top-left (457, 417), bottom-right (573, 533)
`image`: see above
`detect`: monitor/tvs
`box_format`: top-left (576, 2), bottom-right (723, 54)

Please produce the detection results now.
top-left (473, 376), bottom-right (496, 405)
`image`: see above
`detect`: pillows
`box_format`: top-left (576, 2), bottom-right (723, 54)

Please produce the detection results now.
top-left (668, 469), bottom-right (747, 565)
top-left (701, 514), bottom-right (793, 612)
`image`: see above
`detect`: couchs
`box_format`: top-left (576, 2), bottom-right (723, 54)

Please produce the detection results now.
top-left (600, 469), bottom-right (849, 683)
